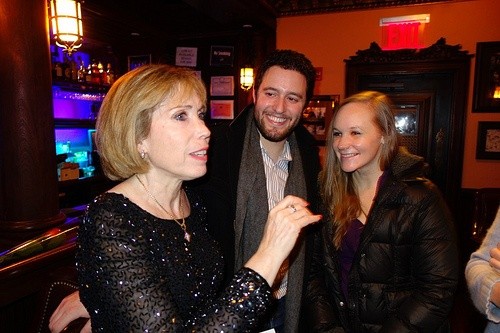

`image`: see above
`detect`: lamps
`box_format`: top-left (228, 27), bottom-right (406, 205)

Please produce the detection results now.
top-left (239, 61), bottom-right (253, 96)
top-left (50, 0), bottom-right (83, 55)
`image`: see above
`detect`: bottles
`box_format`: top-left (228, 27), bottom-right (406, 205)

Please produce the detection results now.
top-left (55, 57), bottom-right (117, 90)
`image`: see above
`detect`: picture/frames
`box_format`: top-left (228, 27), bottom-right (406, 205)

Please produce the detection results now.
top-left (300, 95), bottom-right (340, 145)
top-left (475, 121), bottom-right (500, 161)
top-left (472, 41), bottom-right (500, 112)
